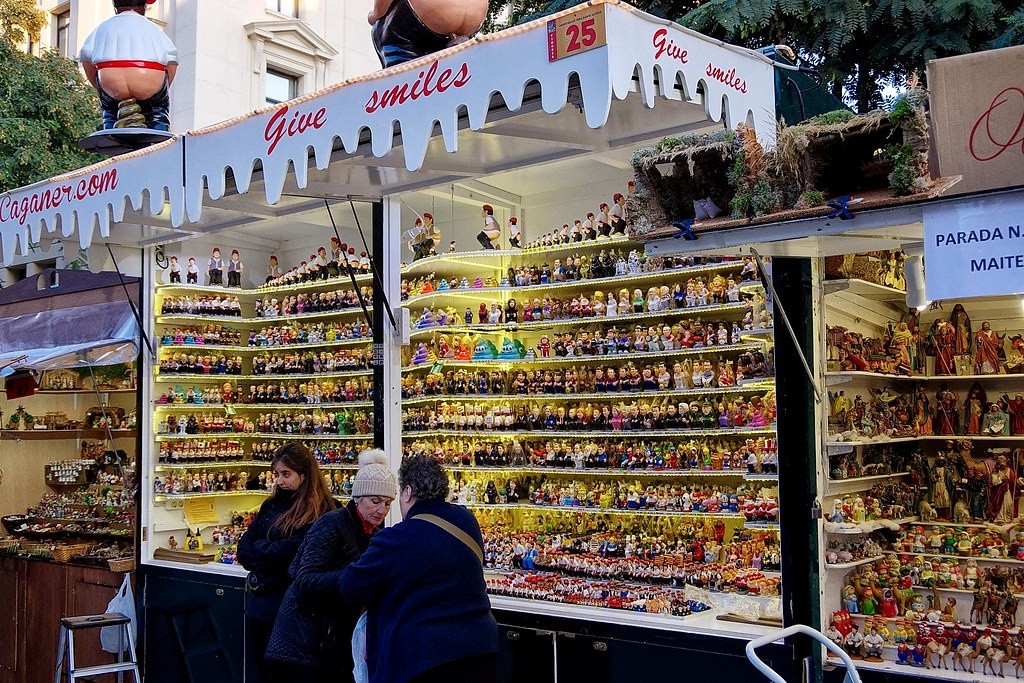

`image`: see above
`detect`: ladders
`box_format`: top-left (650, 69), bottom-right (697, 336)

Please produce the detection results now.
top-left (54, 612), bottom-right (141, 683)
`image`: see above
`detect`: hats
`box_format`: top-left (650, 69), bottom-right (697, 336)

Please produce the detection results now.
top-left (351, 448), bottom-right (397, 500)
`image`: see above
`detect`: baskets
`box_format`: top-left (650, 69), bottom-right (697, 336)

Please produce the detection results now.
top-left (0, 538), bottom-right (86, 563)
top-left (107, 557), bottom-right (134, 572)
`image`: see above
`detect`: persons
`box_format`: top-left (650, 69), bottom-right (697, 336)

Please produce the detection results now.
top-left (78, 0), bottom-right (183, 131)
top-left (827, 251), bottom-right (1024, 682)
top-left (367, 0), bottom-right (491, 73)
top-left (259, 445), bottom-right (400, 682)
top-left (404, 180), bottom-right (781, 621)
top-left (155, 235), bottom-right (375, 566)
top-left (232, 440), bottom-right (349, 682)
top-left (337, 453), bottom-right (504, 682)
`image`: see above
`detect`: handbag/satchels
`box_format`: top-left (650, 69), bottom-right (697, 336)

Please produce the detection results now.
top-left (100, 573), bottom-right (137, 654)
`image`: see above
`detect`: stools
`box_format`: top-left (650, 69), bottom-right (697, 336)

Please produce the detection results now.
top-left (55, 612), bottom-right (140, 683)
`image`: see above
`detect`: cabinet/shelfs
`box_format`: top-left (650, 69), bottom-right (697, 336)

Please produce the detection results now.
top-left (150, 224), bottom-right (1024, 683)
top-left (0, 383), bottom-right (137, 683)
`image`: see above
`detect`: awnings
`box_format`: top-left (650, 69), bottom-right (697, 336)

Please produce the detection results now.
top-left (0, 0), bottom-right (790, 275)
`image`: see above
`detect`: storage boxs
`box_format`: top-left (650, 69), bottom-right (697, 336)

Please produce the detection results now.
top-left (924, 45), bottom-right (1023, 196)
